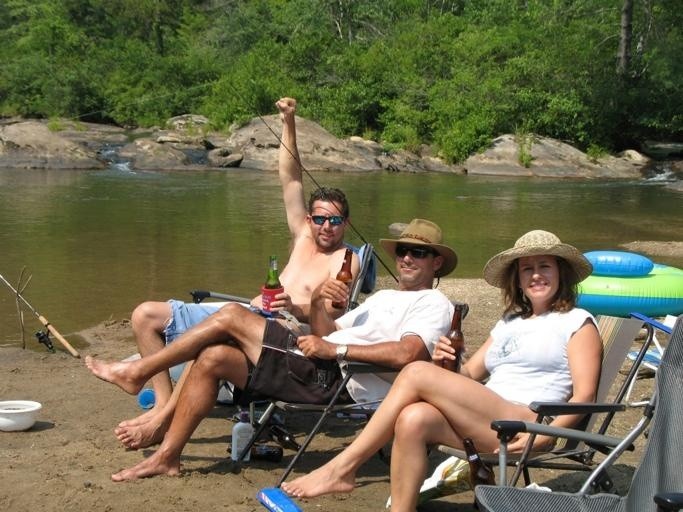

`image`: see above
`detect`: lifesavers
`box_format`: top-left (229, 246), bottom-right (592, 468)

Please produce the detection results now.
top-left (571, 263), bottom-right (683, 319)
top-left (582, 251), bottom-right (656, 277)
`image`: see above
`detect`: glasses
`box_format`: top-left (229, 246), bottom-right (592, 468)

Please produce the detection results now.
top-left (309, 213), bottom-right (344, 227)
top-left (390, 242), bottom-right (436, 259)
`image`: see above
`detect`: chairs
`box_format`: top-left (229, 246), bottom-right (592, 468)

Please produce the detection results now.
top-left (231, 304), bottom-right (469, 494)
top-left (625, 312), bottom-right (678, 408)
top-left (384, 312), bottom-right (650, 512)
top-left (189, 240), bottom-right (374, 421)
top-left (474, 313), bottom-right (683, 512)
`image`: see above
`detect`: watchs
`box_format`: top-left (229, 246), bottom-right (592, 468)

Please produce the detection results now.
top-left (335, 340), bottom-right (350, 360)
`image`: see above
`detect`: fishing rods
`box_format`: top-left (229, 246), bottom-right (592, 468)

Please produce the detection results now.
top-left (0, 275), bottom-right (80, 359)
top-left (224, 82), bottom-right (400, 285)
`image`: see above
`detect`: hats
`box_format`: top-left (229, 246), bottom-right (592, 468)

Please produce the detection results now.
top-left (378, 218), bottom-right (458, 279)
top-left (480, 229), bottom-right (594, 287)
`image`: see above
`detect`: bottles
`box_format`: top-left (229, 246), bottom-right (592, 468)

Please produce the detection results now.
top-left (442, 302), bottom-right (463, 374)
top-left (329, 247), bottom-right (355, 309)
top-left (229, 425), bottom-right (305, 461)
top-left (463, 438), bottom-right (495, 488)
top-left (260, 254), bottom-right (283, 317)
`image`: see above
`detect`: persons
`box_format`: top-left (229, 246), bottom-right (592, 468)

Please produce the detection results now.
top-left (83, 218), bottom-right (458, 484)
top-left (278, 227), bottom-right (603, 511)
top-left (113, 96), bottom-right (361, 452)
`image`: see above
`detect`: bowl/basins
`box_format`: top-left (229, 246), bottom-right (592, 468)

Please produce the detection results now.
top-left (1, 400), bottom-right (42, 432)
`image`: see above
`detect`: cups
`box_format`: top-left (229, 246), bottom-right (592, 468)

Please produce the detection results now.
top-left (139, 389), bottom-right (155, 408)
